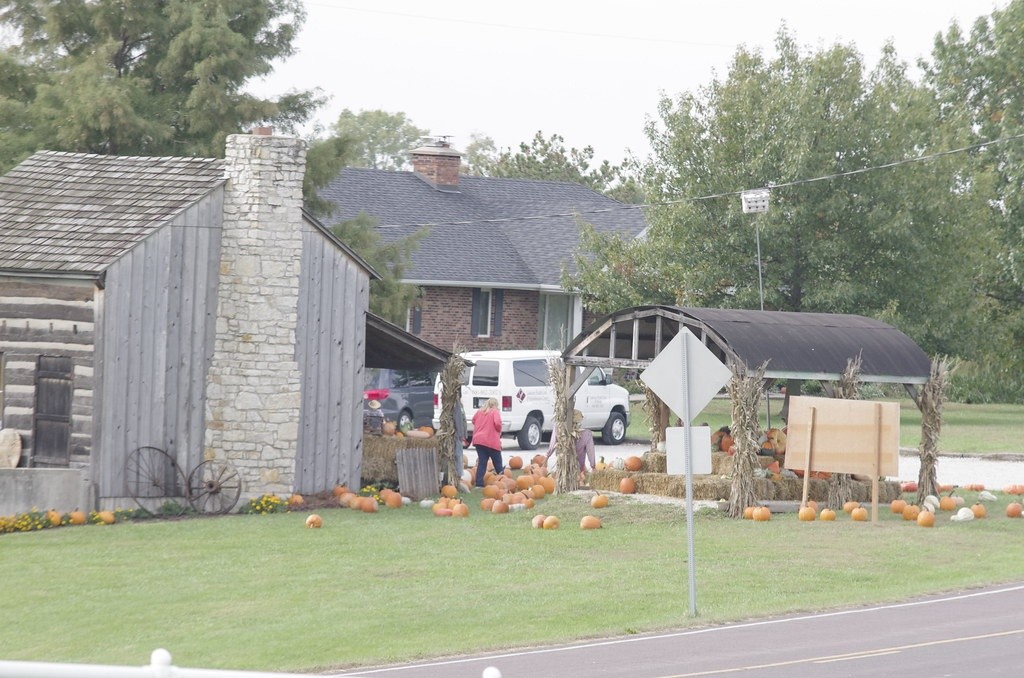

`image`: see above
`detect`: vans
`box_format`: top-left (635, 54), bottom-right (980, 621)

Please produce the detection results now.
top-left (430, 349), bottom-right (632, 450)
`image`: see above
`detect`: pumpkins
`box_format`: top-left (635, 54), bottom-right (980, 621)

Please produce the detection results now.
top-left (288, 421), bottom-right (1024, 531)
top-left (0, 506), bottom-right (116, 536)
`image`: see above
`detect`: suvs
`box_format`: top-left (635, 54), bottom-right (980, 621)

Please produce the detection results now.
top-left (363, 363), bottom-right (436, 432)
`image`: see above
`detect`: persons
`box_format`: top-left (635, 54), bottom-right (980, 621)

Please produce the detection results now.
top-left (442, 391), bottom-right (467, 489)
top-left (472, 397), bottom-right (506, 488)
